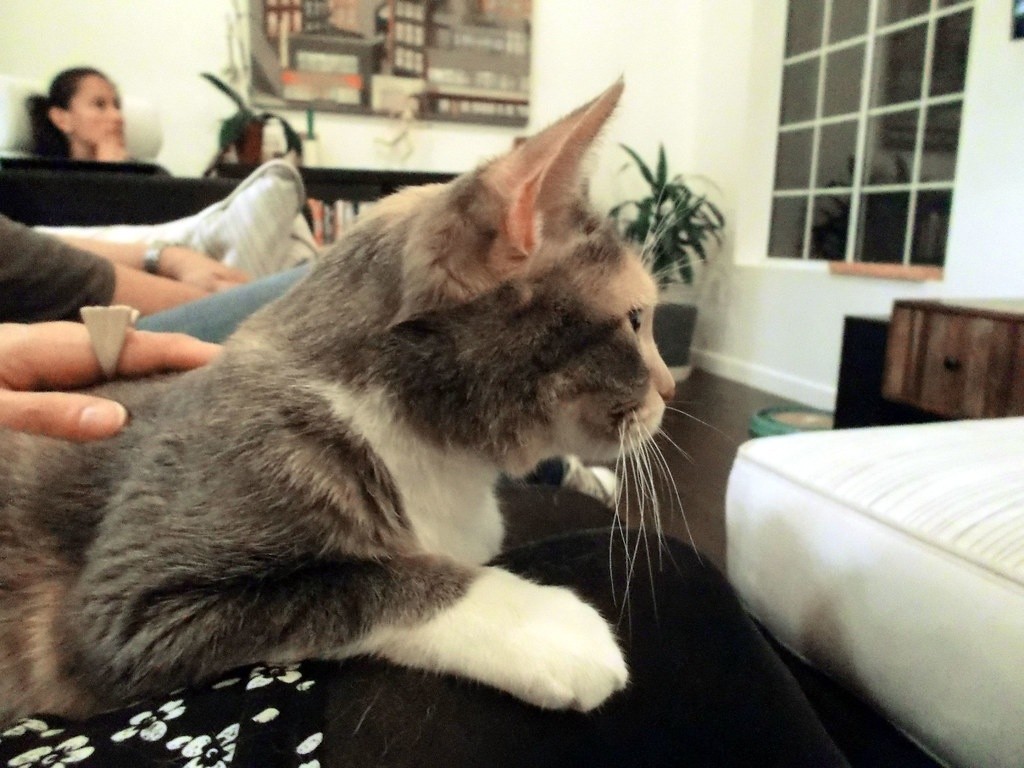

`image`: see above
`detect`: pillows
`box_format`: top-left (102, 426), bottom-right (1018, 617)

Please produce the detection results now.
top-left (26, 158), bottom-right (319, 284)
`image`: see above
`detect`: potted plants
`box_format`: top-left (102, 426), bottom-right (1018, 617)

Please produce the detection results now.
top-left (606, 141), bottom-right (725, 362)
top-left (197, 72), bottom-right (304, 178)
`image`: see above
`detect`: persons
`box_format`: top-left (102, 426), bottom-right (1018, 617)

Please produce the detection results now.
top-left (1, 319), bottom-right (225, 443)
top-left (0, 213), bottom-right (624, 513)
top-left (26, 67), bottom-right (129, 164)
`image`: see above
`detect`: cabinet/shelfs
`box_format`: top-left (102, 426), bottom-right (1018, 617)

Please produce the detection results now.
top-left (883, 294), bottom-right (1024, 419)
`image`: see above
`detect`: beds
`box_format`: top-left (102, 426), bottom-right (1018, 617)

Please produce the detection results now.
top-left (718, 413), bottom-right (1022, 766)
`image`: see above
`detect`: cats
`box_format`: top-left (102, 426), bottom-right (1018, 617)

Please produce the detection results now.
top-left (0, 70), bottom-right (738, 715)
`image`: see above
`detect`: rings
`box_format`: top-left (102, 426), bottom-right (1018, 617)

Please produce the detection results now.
top-left (79, 303), bottom-right (142, 383)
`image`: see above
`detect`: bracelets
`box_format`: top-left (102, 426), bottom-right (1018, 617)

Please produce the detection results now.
top-left (143, 241), bottom-right (168, 275)
